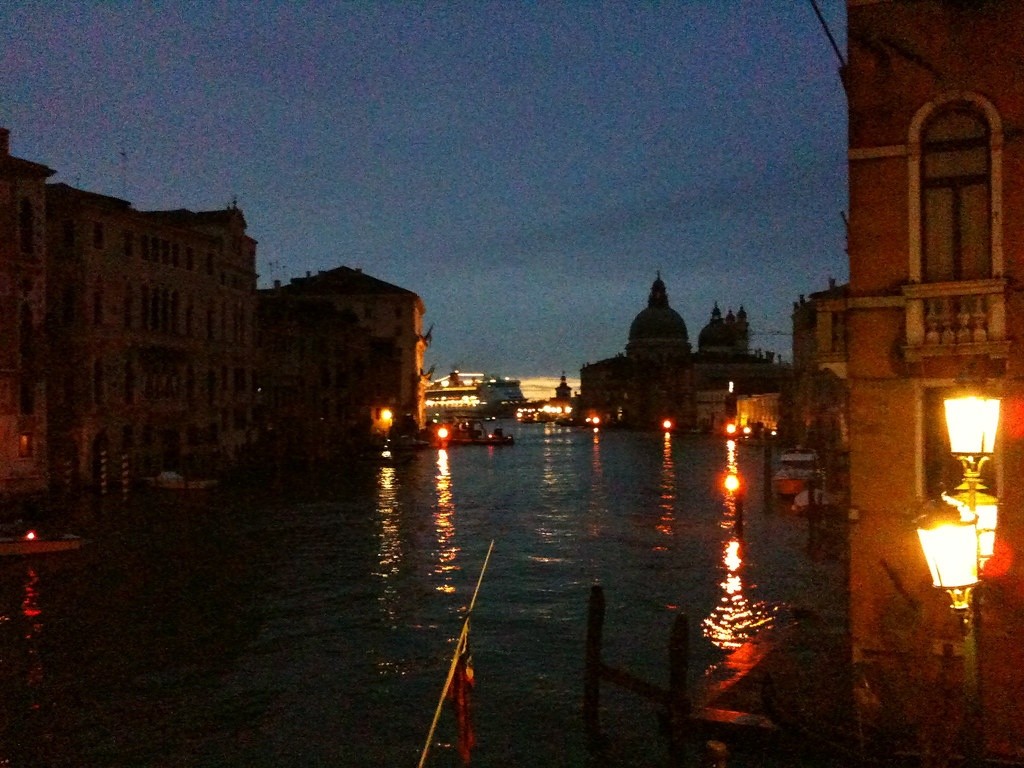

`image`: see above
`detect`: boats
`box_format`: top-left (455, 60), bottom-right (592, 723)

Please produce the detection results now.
top-left (1, 520), bottom-right (92, 559)
top-left (440, 403), bottom-right (603, 449)
